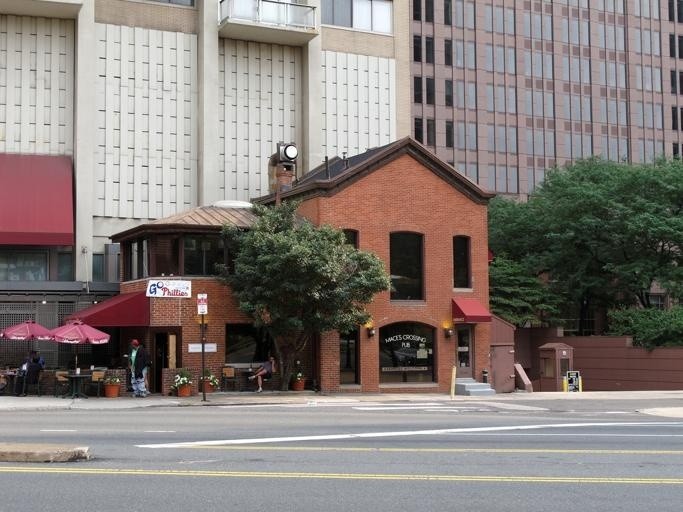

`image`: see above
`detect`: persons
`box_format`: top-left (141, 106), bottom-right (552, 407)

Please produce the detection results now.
top-left (128, 339), bottom-right (146, 398)
top-left (247, 356), bottom-right (279, 393)
top-left (140, 343), bottom-right (151, 395)
top-left (14, 355), bottom-right (43, 397)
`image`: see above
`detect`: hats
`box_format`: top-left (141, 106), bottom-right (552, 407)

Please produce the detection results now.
top-left (131, 339), bottom-right (138, 345)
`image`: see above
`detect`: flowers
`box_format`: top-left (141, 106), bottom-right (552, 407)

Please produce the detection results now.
top-left (291, 371), bottom-right (304, 383)
top-left (104, 375), bottom-right (123, 384)
top-left (201, 368), bottom-right (219, 388)
top-left (170, 368), bottom-right (193, 392)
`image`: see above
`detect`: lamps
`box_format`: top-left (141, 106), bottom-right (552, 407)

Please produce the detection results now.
top-left (444, 328), bottom-right (453, 338)
top-left (367, 326), bottom-right (375, 337)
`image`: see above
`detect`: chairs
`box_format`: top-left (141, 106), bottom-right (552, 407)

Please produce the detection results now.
top-left (82, 370), bottom-right (106, 397)
top-left (22, 366), bottom-right (43, 396)
top-left (54, 371), bottom-right (69, 398)
top-left (220, 366), bottom-right (240, 393)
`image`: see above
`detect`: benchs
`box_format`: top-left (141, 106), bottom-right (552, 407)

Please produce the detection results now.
top-left (252, 371), bottom-right (281, 391)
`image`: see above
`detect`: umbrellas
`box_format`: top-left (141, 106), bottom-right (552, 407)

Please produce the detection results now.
top-left (0, 318), bottom-right (54, 364)
top-left (49, 319), bottom-right (111, 375)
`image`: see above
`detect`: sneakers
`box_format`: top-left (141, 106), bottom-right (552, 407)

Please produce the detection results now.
top-left (248, 375), bottom-right (254, 380)
top-left (257, 387), bottom-right (262, 392)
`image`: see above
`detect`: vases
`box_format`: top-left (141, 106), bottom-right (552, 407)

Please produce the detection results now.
top-left (105, 384), bottom-right (120, 398)
top-left (178, 383), bottom-right (192, 396)
top-left (293, 379), bottom-right (304, 390)
top-left (202, 380), bottom-right (214, 393)
